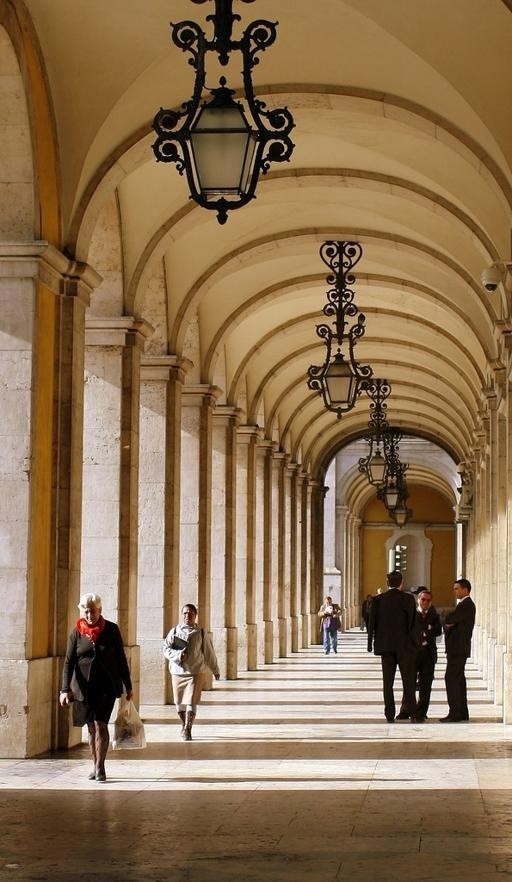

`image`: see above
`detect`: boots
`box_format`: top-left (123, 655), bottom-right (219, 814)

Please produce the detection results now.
top-left (177, 710), bottom-right (196, 742)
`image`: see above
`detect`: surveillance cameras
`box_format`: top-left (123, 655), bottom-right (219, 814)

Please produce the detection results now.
top-left (481, 267), bottom-right (500, 293)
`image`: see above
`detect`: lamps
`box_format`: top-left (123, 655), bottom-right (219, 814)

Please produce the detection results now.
top-left (308, 234), bottom-right (375, 420)
top-left (150, 2), bottom-right (299, 223)
top-left (360, 375), bottom-right (412, 531)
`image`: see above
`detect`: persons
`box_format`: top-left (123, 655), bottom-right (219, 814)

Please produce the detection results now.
top-left (162, 604), bottom-right (221, 740)
top-left (318, 596), bottom-right (343, 655)
top-left (362, 571), bottom-right (476, 722)
top-left (59, 593), bottom-right (134, 783)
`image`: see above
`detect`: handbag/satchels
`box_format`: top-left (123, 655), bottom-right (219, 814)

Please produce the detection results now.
top-left (326, 618), bottom-right (342, 630)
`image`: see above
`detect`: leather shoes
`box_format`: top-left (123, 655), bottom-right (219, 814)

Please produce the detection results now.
top-left (438, 715), bottom-right (464, 722)
top-left (464, 714), bottom-right (470, 721)
top-left (87, 769), bottom-right (96, 781)
top-left (96, 768), bottom-right (107, 782)
top-left (396, 710), bottom-right (428, 723)
top-left (385, 714), bottom-right (396, 723)
